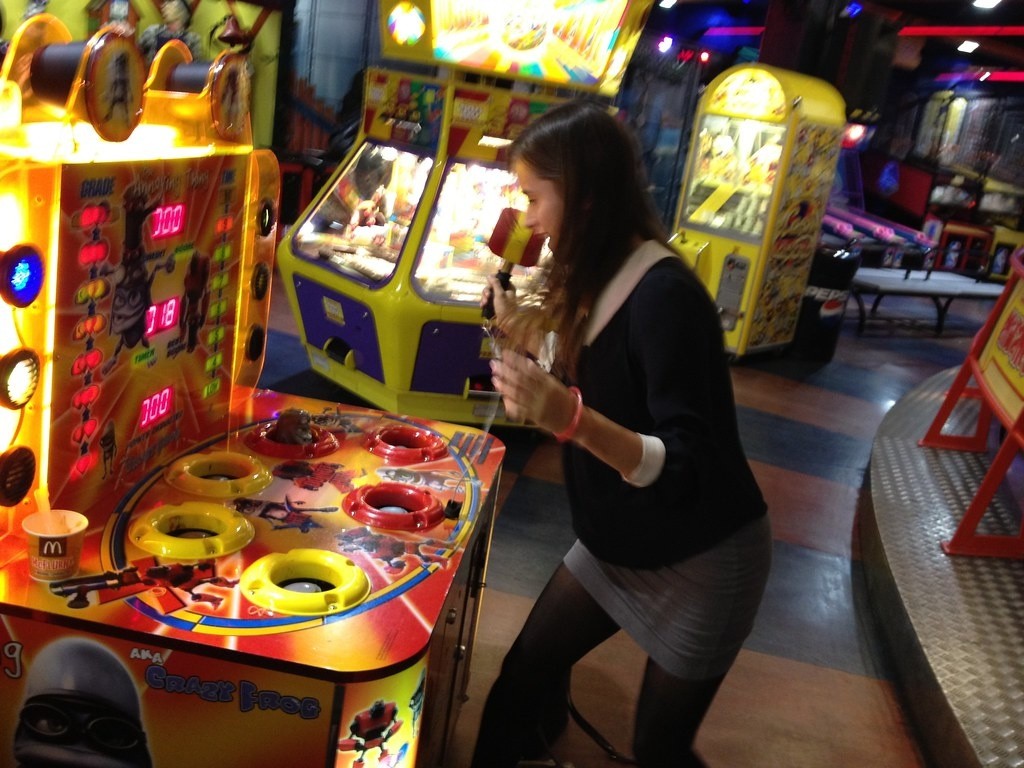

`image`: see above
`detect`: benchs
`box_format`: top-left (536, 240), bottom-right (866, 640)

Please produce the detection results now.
top-left (851, 268), bottom-right (1005, 332)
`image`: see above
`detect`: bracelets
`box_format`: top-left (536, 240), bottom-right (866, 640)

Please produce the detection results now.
top-left (552, 384), bottom-right (583, 443)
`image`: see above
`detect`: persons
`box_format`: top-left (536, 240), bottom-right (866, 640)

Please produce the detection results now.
top-left (472, 98), bottom-right (775, 767)
top-left (313, 69), bottom-right (394, 235)
